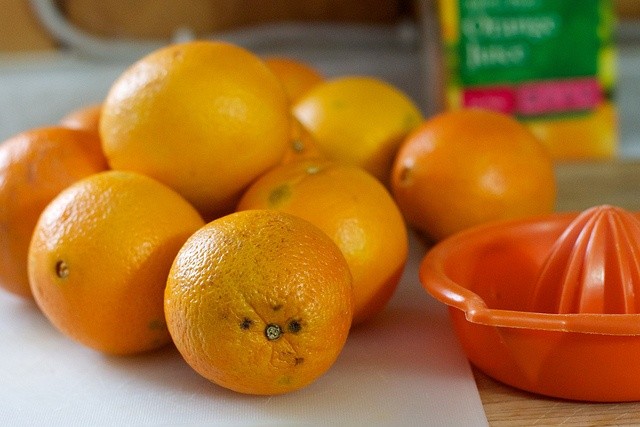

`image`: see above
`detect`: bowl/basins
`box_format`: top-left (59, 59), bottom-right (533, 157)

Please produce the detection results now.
top-left (419, 213), bottom-right (640, 402)
